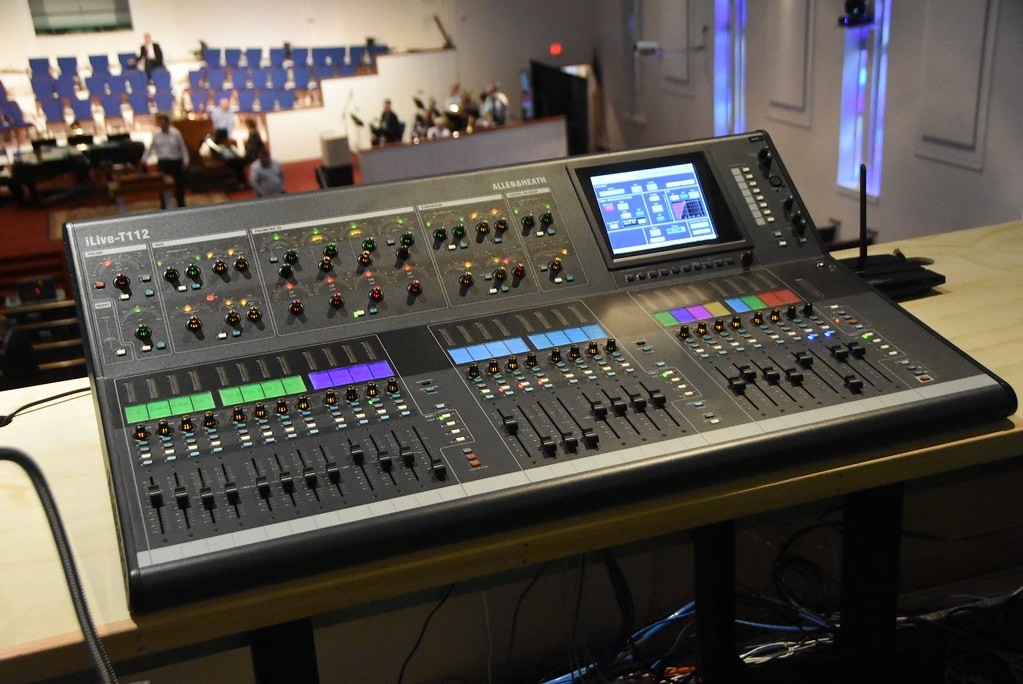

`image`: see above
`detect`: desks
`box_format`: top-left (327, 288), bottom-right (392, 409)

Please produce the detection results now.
top-left (0, 218), bottom-right (1023, 684)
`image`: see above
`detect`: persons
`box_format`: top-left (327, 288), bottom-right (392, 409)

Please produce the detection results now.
top-left (417, 82), bottom-right (509, 140)
top-left (213, 99), bottom-right (284, 197)
top-left (128, 33), bottom-right (164, 83)
top-left (140, 114), bottom-right (189, 210)
top-left (379, 100), bottom-right (400, 145)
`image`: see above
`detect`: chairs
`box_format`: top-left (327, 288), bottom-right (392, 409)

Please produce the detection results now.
top-left (0, 52), bottom-right (175, 148)
top-left (188, 46), bottom-right (388, 117)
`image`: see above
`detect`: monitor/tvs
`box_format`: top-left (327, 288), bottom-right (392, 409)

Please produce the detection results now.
top-left (565, 144), bottom-right (758, 273)
